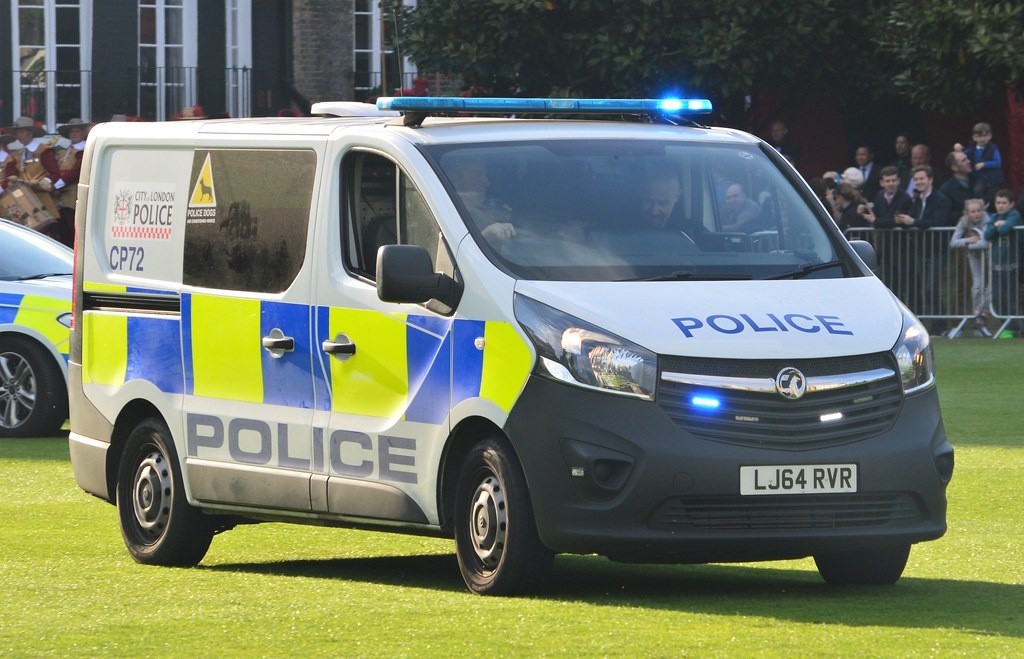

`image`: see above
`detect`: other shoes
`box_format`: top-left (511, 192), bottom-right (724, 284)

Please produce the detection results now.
top-left (973, 325), bottom-right (992, 336)
top-left (998, 329), bottom-right (1018, 339)
top-left (929, 323), bottom-right (948, 337)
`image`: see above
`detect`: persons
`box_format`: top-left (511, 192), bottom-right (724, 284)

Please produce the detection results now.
top-left (448, 155), bottom-right (517, 241)
top-left (712, 164), bottom-right (733, 207)
top-left (718, 184), bottom-right (757, 234)
top-left (949, 198), bottom-right (990, 327)
top-left (634, 164), bottom-right (683, 228)
top-left (806, 133), bottom-right (952, 337)
top-left (939, 150), bottom-right (991, 209)
top-left (954, 122), bottom-right (1005, 198)
top-left (766, 120), bottom-right (800, 169)
top-left (0, 114), bottom-right (96, 248)
top-left (985, 189), bottom-right (1024, 337)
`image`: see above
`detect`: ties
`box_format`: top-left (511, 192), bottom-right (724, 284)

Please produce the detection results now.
top-left (862, 167), bottom-right (865, 177)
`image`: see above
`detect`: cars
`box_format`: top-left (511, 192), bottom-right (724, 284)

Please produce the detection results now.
top-left (0, 216), bottom-right (76, 436)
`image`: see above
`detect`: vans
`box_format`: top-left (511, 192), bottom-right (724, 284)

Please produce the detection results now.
top-left (70, 94), bottom-right (952, 601)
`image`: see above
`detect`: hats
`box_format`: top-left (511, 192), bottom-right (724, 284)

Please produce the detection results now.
top-left (5, 117), bottom-right (45, 138)
top-left (110, 113), bottom-right (128, 123)
top-left (58, 117), bottom-right (92, 139)
top-left (174, 105), bottom-right (208, 120)
top-left (841, 167), bottom-right (864, 188)
